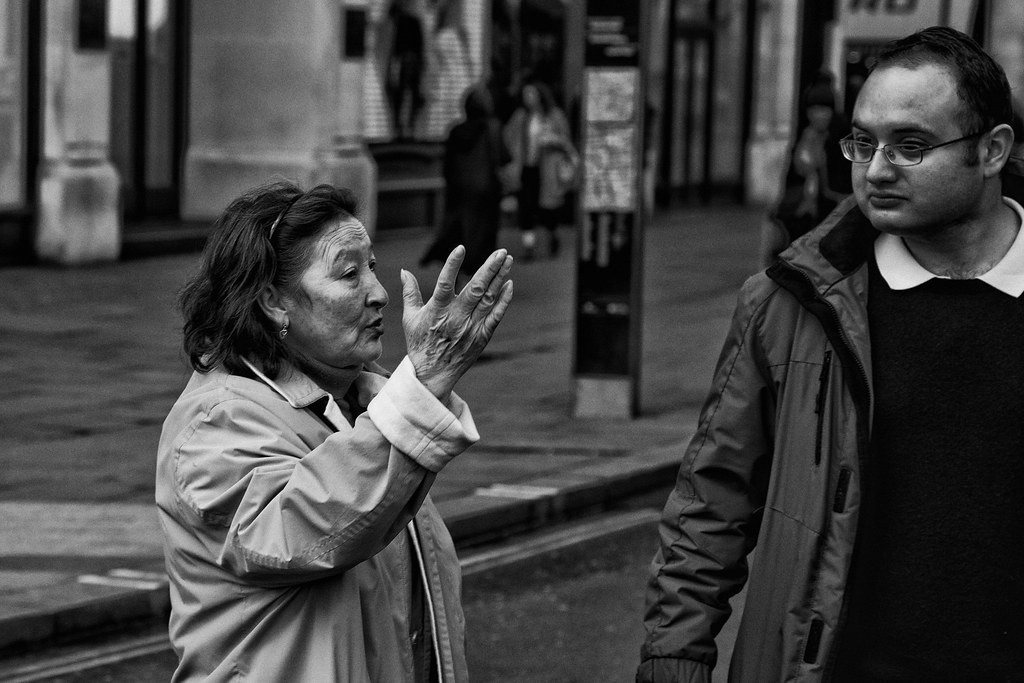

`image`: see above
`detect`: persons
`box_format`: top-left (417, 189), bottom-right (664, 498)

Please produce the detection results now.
top-left (154, 183), bottom-right (516, 683)
top-left (419, 87), bottom-right (514, 275)
top-left (502, 81), bottom-right (577, 263)
top-left (635, 26), bottom-right (1024, 683)
top-left (776, 73), bottom-right (851, 248)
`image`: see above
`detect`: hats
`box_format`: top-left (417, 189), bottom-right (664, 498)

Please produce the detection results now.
top-left (802, 74), bottom-right (835, 109)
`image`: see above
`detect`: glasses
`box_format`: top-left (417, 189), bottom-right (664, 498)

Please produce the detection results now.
top-left (838, 132), bottom-right (981, 167)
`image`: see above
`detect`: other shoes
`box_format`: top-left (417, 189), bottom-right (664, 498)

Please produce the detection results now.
top-left (550, 238), bottom-right (558, 254)
top-left (524, 248), bottom-right (534, 260)
